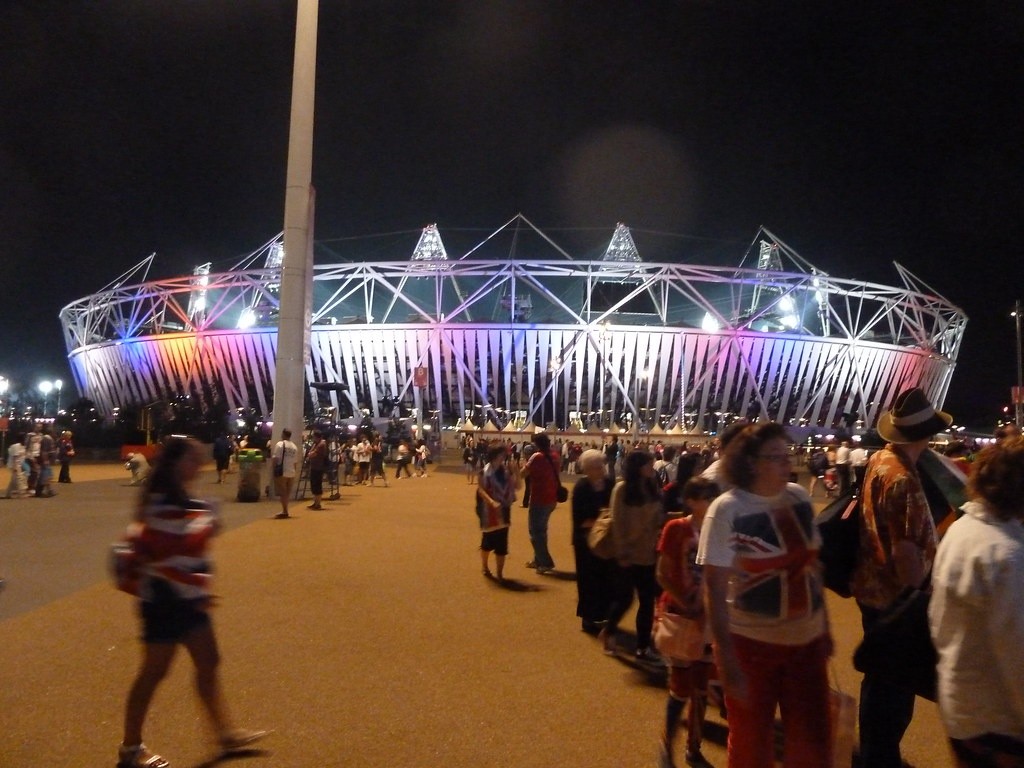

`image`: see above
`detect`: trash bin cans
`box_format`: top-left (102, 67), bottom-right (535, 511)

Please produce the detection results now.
top-left (237, 448), bottom-right (263, 502)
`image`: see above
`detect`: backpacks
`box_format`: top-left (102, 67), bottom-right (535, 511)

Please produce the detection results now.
top-left (422, 445), bottom-right (431, 458)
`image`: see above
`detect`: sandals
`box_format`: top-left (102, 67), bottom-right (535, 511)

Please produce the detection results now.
top-left (117, 742), bottom-right (168, 768)
top-left (218, 725), bottom-right (274, 750)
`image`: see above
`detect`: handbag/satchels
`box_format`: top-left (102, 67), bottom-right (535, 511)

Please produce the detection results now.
top-left (275, 464), bottom-right (284, 477)
top-left (813, 486), bottom-right (860, 598)
top-left (113, 548), bottom-right (138, 595)
top-left (587, 507), bottom-right (616, 560)
top-left (557, 486), bottom-right (568, 503)
top-left (654, 614), bottom-right (703, 661)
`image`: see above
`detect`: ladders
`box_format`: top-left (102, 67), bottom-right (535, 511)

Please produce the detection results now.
top-left (295, 424), bottom-right (341, 501)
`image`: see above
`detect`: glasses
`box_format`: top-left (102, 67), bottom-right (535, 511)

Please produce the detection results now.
top-left (757, 453), bottom-right (792, 465)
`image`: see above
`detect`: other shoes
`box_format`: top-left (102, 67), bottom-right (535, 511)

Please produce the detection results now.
top-left (274, 511), bottom-right (288, 520)
top-left (366, 482), bottom-right (373, 487)
top-left (482, 565), bottom-right (491, 575)
top-left (308, 503), bottom-right (322, 510)
top-left (658, 740), bottom-right (674, 768)
top-left (636, 646), bottom-right (644, 657)
top-left (536, 561), bottom-right (556, 574)
top-left (410, 473), bottom-right (417, 477)
top-left (599, 628), bottom-right (616, 657)
top-left (497, 576), bottom-right (511, 589)
top-left (526, 560), bottom-right (538, 568)
top-left (421, 473), bottom-right (428, 478)
top-left (686, 752), bottom-right (711, 768)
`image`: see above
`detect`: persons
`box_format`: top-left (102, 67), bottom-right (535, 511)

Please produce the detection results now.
top-left (850, 386), bottom-right (1024, 768)
top-left (5, 421), bottom-right (58, 500)
top-left (681, 440), bottom-right (689, 452)
top-left (213, 431), bottom-right (236, 484)
top-left (563, 437), bottom-right (664, 474)
top-left (796, 441), bottom-right (805, 465)
top-left (119, 434), bottom-right (275, 768)
top-left (702, 440), bottom-right (709, 451)
top-left (461, 435), bottom-right (466, 448)
top-left (411, 439), bottom-right (427, 478)
top-left (272, 428), bottom-right (298, 518)
top-left (514, 440), bottom-right (522, 467)
top-left (850, 440), bottom-right (869, 492)
top-left (519, 443), bottom-right (536, 509)
top-left (125, 453), bottom-right (152, 484)
top-left (519, 433), bottom-right (560, 574)
top-left (395, 438), bottom-right (414, 479)
top-left (504, 438), bottom-right (512, 465)
top-left (809, 441), bottom-right (849, 499)
top-left (57, 431), bottom-right (75, 483)
top-left (463, 439), bottom-right (478, 484)
top-left (476, 442), bottom-right (521, 581)
top-left (329, 431), bottom-right (387, 488)
top-left (476, 437), bottom-right (487, 470)
top-left (572, 423), bottom-right (836, 768)
top-left (307, 430), bottom-right (326, 509)
top-left (0, 412), bottom-right (52, 467)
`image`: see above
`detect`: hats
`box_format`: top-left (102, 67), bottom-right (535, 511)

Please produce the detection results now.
top-left (877, 385), bottom-right (953, 444)
